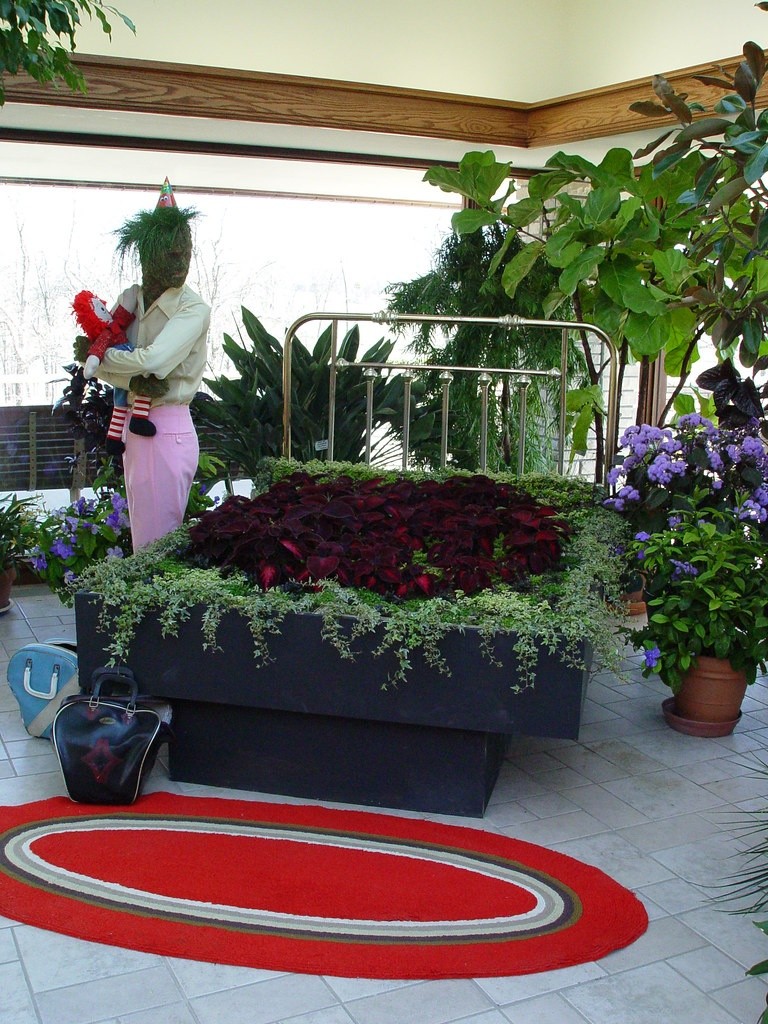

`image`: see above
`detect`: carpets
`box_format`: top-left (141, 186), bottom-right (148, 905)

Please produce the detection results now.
top-left (0, 792), bottom-right (649, 980)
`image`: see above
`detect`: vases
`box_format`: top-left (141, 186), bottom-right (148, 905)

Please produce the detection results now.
top-left (642, 587), bottom-right (660, 621)
top-left (675, 656), bottom-right (747, 722)
top-left (619, 570), bottom-right (648, 602)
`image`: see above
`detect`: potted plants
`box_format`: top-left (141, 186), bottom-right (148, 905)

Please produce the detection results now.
top-left (0, 493), bottom-right (42, 609)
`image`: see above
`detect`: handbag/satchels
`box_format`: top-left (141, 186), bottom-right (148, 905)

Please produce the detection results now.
top-left (6, 637), bottom-right (83, 740)
top-left (52, 664), bottom-right (175, 805)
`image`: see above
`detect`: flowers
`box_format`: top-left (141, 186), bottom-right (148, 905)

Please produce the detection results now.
top-left (29, 451), bottom-right (227, 608)
top-left (603, 413), bottom-right (768, 695)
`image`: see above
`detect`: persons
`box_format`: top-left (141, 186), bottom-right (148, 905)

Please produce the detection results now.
top-left (93, 206), bottom-right (210, 555)
top-left (74, 287), bottom-right (157, 453)
